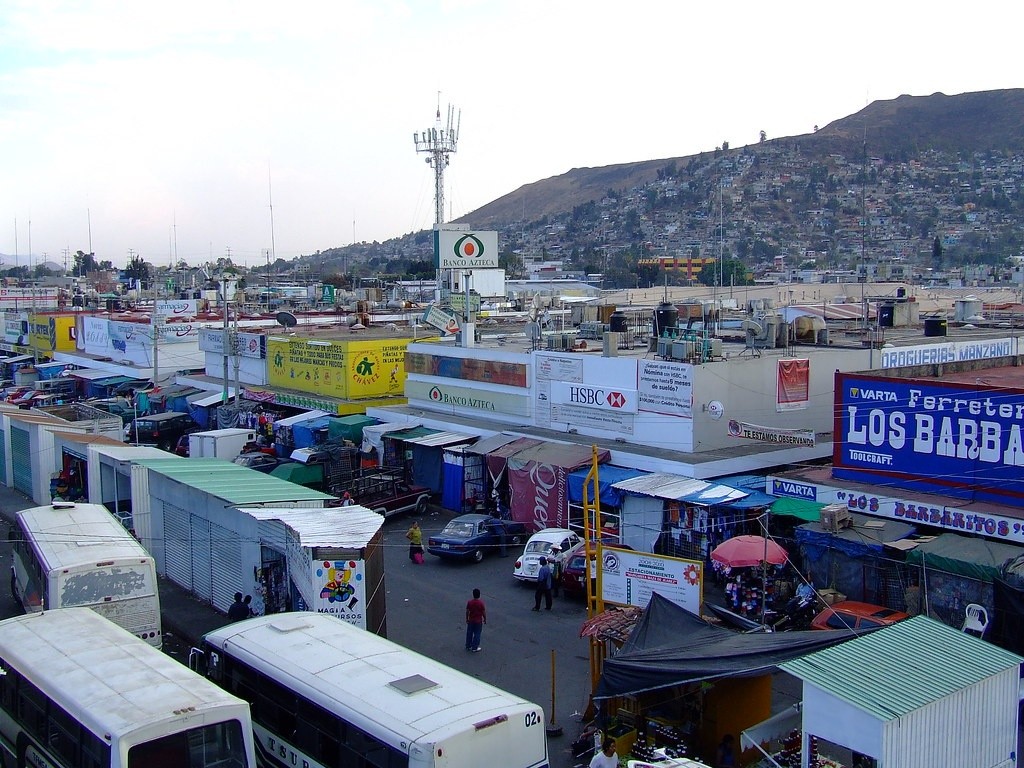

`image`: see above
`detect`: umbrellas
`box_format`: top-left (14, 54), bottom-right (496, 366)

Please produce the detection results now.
top-left (711, 534), bottom-right (789, 567)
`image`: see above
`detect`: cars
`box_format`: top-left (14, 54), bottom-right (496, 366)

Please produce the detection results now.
top-left (231, 451), bottom-right (294, 475)
top-left (122, 422), bottom-right (151, 436)
top-left (810, 600), bottom-right (909, 632)
top-left (427, 513), bottom-right (525, 563)
top-left (513, 528), bottom-right (585, 585)
top-left (563, 541), bottom-right (634, 589)
top-left (0, 381), bottom-right (44, 409)
top-left (176, 435), bottom-right (190, 457)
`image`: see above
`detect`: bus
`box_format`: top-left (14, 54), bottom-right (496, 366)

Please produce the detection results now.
top-left (190, 612), bottom-right (551, 768)
top-left (10, 501), bottom-right (163, 654)
top-left (0, 607), bottom-right (258, 768)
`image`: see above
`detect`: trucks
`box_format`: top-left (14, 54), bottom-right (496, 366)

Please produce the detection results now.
top-left (188, 428), bottom-right (268, 462)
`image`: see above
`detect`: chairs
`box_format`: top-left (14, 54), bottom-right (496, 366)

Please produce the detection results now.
top-left (961, 604), bottom-right (989, 639)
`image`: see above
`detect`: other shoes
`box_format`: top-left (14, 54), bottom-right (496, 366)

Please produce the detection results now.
top-left (545, 607), bottom-right (552, 611)
top-left (530, 607), bottom-right (539, 611)
top-left (412, 560), bottom-right (416, 563)
top-left (472, 647), bottom-right (482, 651)
top-left (466, 646), bottom-right (472, 650)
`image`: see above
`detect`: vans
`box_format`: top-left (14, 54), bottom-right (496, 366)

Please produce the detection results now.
top-left (129, 411), bottom-right (201, 453)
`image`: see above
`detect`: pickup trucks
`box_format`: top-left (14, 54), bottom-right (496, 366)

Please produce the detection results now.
top-left (327, 474), bottom-right (433, 526)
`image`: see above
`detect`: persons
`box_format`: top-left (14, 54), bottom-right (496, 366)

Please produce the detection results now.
top-left (340, 491), bottom-right (354, 506)
top-left (444, 319), bottom-right (455, 335)
top-left (785, 573), bottom-right (815, 625)
top-left (465, 588), bottom-right (486, 652)
top-left (227, 592), bottom-right (252, 622)
top-left (589, 738), bottom-right (619, 768)
top-left (530, 556), bottom-right (552, 612)
top-left (0, 388), bottom-right (10, 401)
top-left (406, 520), bottom-right (425, 563)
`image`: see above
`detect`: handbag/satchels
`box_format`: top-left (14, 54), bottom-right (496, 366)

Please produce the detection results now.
top-left (406, 532), bottom-right (413, 539)
top-left (415, 553), bottom-right (423, 564)
top-left (421, 542), bottom-right (424, 552)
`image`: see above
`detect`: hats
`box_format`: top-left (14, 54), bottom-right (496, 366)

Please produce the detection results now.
top-left (551, 542), bottom-right (562, 549)
top-left (344, 492), bottom-right (350, 499)
top-left (537, 556), bottom-right (545, 561)
